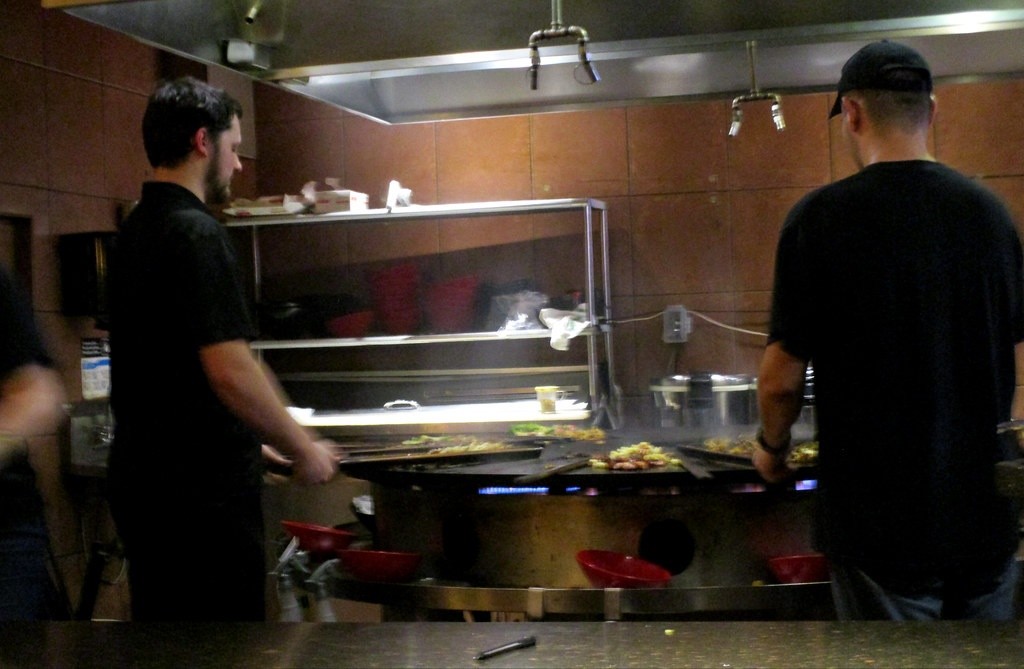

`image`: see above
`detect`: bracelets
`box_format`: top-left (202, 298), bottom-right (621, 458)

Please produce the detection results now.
top-left (754, 425), bottom-right (793, 455)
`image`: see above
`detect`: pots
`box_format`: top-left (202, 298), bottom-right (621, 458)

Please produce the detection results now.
top-left (647, 370), bottom-right (760, 434)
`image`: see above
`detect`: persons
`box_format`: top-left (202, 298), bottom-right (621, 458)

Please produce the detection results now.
top-left (0, 264), bottom-right (73, 624)
top-left (752, 39), bottom-right (1024, 627)
top-left (104, 74), bottom-right (349, 621)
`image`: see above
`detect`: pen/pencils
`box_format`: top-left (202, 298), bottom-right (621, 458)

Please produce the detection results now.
top-left (473, 636), bottom-right (537, 660)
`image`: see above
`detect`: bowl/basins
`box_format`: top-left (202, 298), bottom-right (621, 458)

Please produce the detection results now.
top-left (338, 549), bottom-right (421, 582)
top-left (323, 261), bottom-right (606, 337)
top-left (769, 554), bottom-right (826, 584)
top-left (278, 520), bottom-right (351, 551)
top-left (576, 550), bottom-right (672, 588)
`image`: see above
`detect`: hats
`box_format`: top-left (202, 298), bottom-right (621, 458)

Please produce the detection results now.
top-left (828, 40), bottom-right (935, 118)
